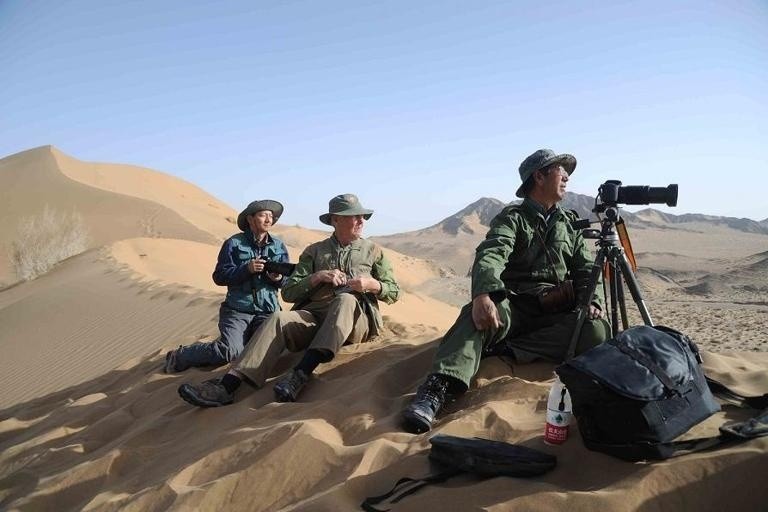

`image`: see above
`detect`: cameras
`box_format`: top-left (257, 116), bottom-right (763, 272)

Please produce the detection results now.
top-left (259, 255), bottom-right (295, 276)
top-left (595, 181), bottom-right (679, 213)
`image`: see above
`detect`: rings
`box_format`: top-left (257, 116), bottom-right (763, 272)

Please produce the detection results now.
top-left (339, 274), bottom-right (342, 278)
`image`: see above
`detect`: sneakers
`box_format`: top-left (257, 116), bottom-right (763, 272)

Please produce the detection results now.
top-left (178, 376), bottom-right (234, 408)
top-left (405, 375), bottom-right (448, 430)
top-left (274, 368), bottom-right (310, 401)
top-left (164, 350), bottom-right (180, 374)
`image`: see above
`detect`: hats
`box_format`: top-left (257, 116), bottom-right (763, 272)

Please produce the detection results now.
top-left (515, 149), bottom-right (576, 198)
top-left (238, 199), bottom-right (283, 231)
top-left (320, 193), bottom-right (374, 224)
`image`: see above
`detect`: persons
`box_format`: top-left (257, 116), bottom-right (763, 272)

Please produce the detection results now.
top-left (402, 149), bottom-right (613, 433)
top-left (164, 200), bottom-right (289, 375)
top-left (179, 194), bottom-right (400, 408)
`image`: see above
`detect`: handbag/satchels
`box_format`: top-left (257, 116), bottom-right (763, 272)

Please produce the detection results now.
top-left (556, 325), bottom-right (722, 462)
top-left (429, 434), bottom-right (556, 477)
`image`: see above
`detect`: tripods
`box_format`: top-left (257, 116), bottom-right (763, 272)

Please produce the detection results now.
top-left (567, 229), bottom-right (655, 356)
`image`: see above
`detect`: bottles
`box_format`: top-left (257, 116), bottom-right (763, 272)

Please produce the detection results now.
top-left (545, 378), bottom-right (572, 445)
